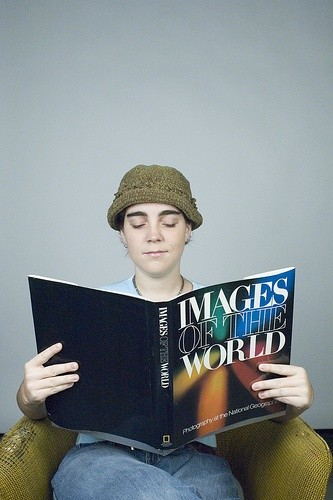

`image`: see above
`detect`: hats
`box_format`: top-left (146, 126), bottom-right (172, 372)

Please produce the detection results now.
top-left (106, 165), bottom-right (203, 231)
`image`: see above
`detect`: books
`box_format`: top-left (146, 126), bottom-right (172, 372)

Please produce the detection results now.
top-left (26, 267), bottom-right (296, 456)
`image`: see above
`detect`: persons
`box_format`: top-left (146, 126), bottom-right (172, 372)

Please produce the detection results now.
top-left (16, 164), bottom-right (313, 500)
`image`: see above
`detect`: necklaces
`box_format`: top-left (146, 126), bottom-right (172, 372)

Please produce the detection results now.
top-left (133, 274), bottom-right (184, 297)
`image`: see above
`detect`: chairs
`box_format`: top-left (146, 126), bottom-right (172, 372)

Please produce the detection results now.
top-left (0, 414), bottom-right (333, 500)
